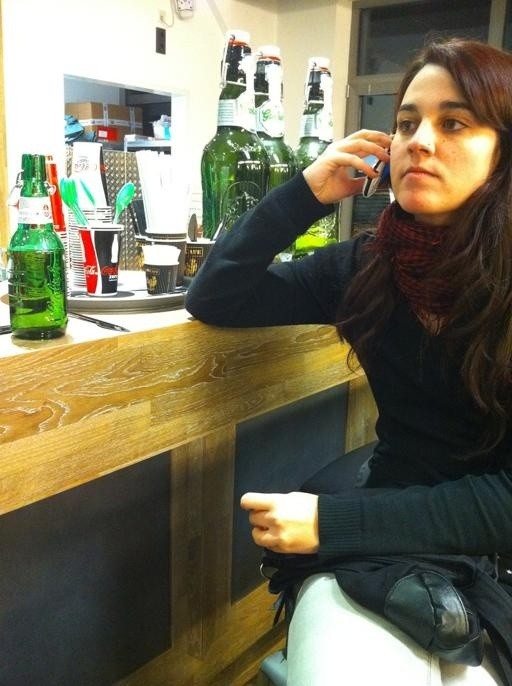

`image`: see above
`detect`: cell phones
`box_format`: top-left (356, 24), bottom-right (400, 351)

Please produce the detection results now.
top-left (362, 134), bottom-right (395, 198)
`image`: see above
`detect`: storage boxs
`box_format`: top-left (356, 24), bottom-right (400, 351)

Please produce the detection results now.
top-left (65, 102), bottom-right (143, 144)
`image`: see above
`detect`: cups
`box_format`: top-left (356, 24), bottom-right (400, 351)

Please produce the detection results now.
top-left (67, 203), bottom-right (125, 297)
top-left (144, 233), bottom-right (215, 294)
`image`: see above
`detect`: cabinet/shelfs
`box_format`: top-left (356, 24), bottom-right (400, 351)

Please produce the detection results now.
top-left (1, 321), bottom-right (380, 685)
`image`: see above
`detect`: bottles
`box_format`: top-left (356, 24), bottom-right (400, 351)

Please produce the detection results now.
top-left (294, 54), bottom-right (341, 258)
top-left (196, 28), bottom-right (301, 256)
top-left (5, 153), bottom-right (70, 343)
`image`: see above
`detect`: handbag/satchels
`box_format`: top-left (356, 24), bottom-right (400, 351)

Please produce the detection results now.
top-left (261, 547), bottom-right (498, 665)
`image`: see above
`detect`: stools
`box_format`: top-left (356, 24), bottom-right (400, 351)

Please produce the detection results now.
top-left (257, 650), bottom-right (289, 686)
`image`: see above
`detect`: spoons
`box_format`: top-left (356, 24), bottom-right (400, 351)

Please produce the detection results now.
top-left (59, 178), bottom-right (136, 225)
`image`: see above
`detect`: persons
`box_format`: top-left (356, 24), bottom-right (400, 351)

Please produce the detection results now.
top-left (184, 38), bottom-right (512, 686)
